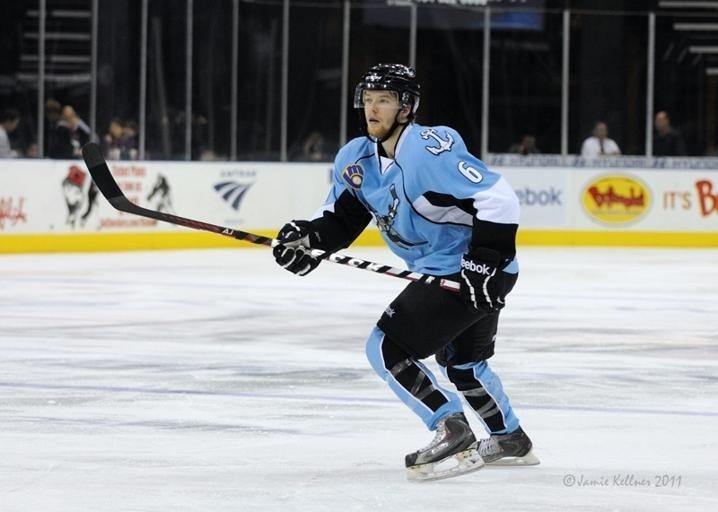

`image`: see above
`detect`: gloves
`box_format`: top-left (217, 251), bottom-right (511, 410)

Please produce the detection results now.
top-left (271, 218), bottom-right (325, 277)
top-left (459, 252), bottom-right (516, 314)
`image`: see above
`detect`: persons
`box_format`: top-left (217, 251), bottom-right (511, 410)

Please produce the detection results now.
top-left (293, 129), bottom-right (334, 161)
top-left (273, 64), bottom-right (532, 467)
top-left (653, 110), bottom-right (686, 155)
top-left (0, 98), bottom-right (140, 160)
top-left (579, 122), bottom-right (622, 155)
top-left (508, 121), bottom-right (541, 153)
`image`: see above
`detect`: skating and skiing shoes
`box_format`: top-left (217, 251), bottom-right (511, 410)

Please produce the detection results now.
top-left (405, 415), bottom-right (542, 468)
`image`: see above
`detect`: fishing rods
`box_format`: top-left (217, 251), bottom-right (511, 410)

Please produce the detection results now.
top-left (82, 144), bottom-right (460, 291)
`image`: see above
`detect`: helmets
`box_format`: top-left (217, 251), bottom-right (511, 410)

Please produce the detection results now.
top-left (353, 64), bottom-right (422, 116)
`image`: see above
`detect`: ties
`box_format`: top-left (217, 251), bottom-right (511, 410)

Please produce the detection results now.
top-left (599, 138), bottom-right (604, 154)
